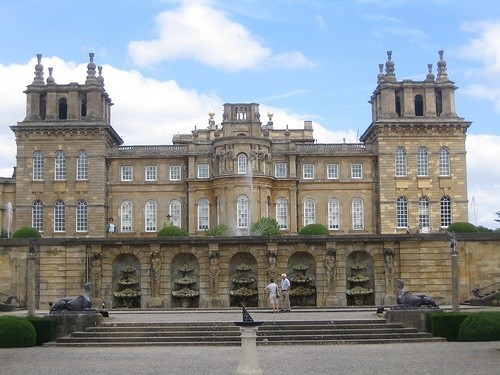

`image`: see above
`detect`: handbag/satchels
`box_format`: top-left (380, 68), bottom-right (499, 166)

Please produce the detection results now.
top-left (276, 291), bottom-right (279, 299)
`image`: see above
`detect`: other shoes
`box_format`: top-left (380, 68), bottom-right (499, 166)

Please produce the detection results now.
top-left (274, 312), bottom-right (275, 314)
top-left (277, 312), bottom-right (279, 314)
top-left (283, 308), bottom-right (290, 311)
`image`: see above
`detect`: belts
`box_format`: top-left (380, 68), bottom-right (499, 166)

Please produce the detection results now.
top-left (282, 290), bottom-right (287, 291)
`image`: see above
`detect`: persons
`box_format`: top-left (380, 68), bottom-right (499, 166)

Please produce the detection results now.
top-left (105, 217), bottom-right (116, 237)
top-left (91, 251), bottom-right (103, 298)
top-left (163, 214), bottom-right (174, 228)
top-left (278, 273), bottom-right (291, 312)
top-left (267, 249), bottom-right (278, 284)
top-left (395, 278), bottom-right (438, 306)
top-left (148, 250), bottom-right (162, 298)
top-left (325, 248), bottom-right (336, 296)
top-left (208, 250), bottom-right (221, 299)
top-left (383, 248), bottom-right (395, 295)
top-left (50, 281), bottom-right (93, 311)
top-left (264, 278), bottom-right (280, 314)
